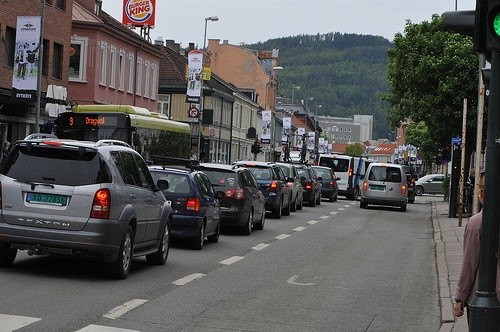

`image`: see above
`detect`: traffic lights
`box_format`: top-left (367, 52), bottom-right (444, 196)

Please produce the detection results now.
top-left (481, 67), bottom-right (493, 90)
top-left (474, 1), bottom-right (500, 46)
top-left (441, 10), bottom-right (475, 35)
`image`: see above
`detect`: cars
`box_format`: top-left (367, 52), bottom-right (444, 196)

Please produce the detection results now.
top-left (390, 173), bottom-right (417, 203)
top-left (276, 162), bottom-right (306, 212)
top-left (415, 174), bottom-right (446, 197)
top-left (311, 165), bottom-right (341, 202)
top-left (359, 162), bottom-right (408, 212)
top-left (140, 165), bottom-right (226, 251)
top-left (291, 160), bottom-right (324, 208)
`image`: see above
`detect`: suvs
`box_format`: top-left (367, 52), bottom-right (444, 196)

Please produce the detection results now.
top-left (0, 133), bottom-right (174, 280)
top-left (200, 162), bottom-right (270, 236)
top-left (232, 161), bottom-right (296, 220)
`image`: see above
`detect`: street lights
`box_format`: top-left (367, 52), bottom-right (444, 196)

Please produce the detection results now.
top-left (315, 105), bottom-right (323, 130)
top-left (291, 86), bottom-right (300, 113)
top-left (197, 16), bottom-right (219, 159)
top-left (271, 66), bottom-right (284, 162)
top-left (304, 97), bottom-right (313, 129)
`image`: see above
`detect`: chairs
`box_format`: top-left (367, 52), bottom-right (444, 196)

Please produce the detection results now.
top-left (174, 184), bottom-right (187, 192)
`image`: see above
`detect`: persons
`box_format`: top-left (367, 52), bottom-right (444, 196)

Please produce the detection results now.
top-left (454, 170), bottom-right (500, 332)
top-left (3, 141), bottom-right (10, 160)
top-left (263, 121), bottom-right (271, 135)
top-left (189, 70), bottom-right (196, 89)
top-left (420, 171), bottom-right (424, 176)
top-left (443, 174), bottom-right (450, 201)
top-left (465, 168), bottom-right (475, 213)
top-left (17, 43), bottom-right (39, 81)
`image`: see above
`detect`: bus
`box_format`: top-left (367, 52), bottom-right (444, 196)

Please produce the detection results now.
top-left (40, 104), bottom-right (191, 167)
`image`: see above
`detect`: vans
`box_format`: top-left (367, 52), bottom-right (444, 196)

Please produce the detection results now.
top-left (317, 153), bottom-right (378, 201)
